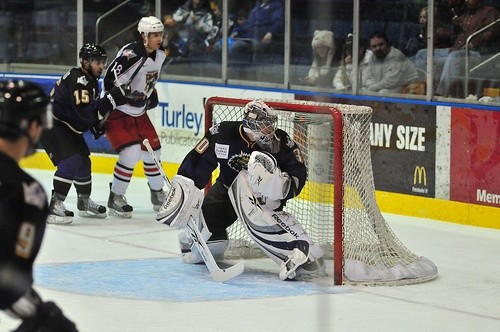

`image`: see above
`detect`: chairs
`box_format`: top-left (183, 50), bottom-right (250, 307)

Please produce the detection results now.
top-left (172, 11), bottom-right (423, 87)
top-left (0, 0), bottom-right (133, 59)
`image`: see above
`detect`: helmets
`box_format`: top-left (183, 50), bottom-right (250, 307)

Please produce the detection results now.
top-left (79, 43), bottom-right (107, 59)
top-left (243, 100), bottom-right (278, 144)
top-left (137, 16), bottom-right (164, 36)
top-left (0, 80), bottom-right (49, 111)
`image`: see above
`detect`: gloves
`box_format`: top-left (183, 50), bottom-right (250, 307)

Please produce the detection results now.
top-left (145, 89), bottom-right (159, 110)
top-left (106, 84), bottom-right (130, 109)
top-left (29, 299), bottom-right (79, 331)
top-left (128, 90), bottom-right (147, 107)
top-left (90, 124), bottom-right (105, 139)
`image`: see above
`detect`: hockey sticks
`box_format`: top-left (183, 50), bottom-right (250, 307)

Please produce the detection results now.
top-left (96, 30), bottom-right (149, 131)
top-left (114, 69), bottom-right (148, 101)
top-left (143, 139), bottom-right (246, 282)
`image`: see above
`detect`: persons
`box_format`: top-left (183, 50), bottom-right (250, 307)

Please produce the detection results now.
top-left (98, 16), bottom-right (168, 217)
top-left (41, 44), bottom-right (129, 225)
top-left (156, 101), bottom-right (327, 279)
top-left (0, 79), bottom-right (78, 332)
top-left (410, 0), bottom-right (500, 99)
top-left (135, 0), bottom-right (286, 61)
top-left (305, 30), bottom-right (419, 94)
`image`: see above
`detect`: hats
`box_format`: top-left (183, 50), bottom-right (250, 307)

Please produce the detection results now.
top-left (308, 30), bottom-right (335, 80)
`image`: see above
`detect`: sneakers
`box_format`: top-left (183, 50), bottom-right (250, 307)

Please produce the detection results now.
top-left (147, 182), bottom-right (169, 211)
top-left (77, 191), bottom-right (107, 218)
top-left (46, 190), bottom-right (74, 224)
top-left (108, 182), bottom-right (133, 217)
